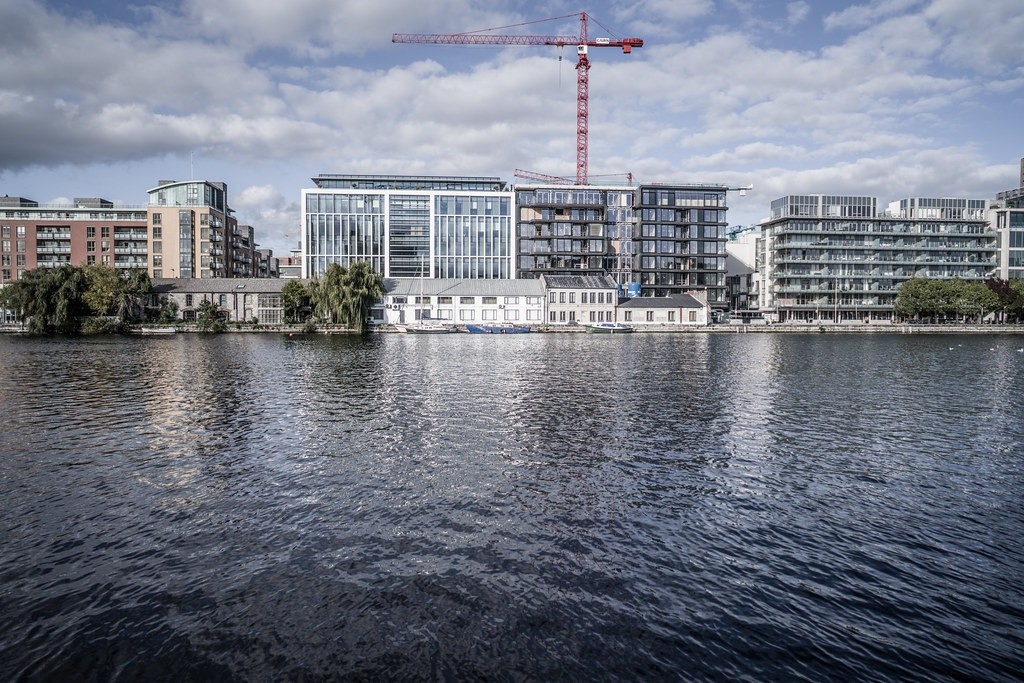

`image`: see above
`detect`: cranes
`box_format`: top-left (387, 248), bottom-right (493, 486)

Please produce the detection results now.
top-left (391, 9), bottom-right (645, 184)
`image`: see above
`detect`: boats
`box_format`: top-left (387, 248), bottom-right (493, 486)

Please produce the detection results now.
top-left (466, 322), bottom-right (532, 334)
top-left (395, 251), bottom-right (459, 333)
top-left (584, 323), bottom-right (638, 334)
